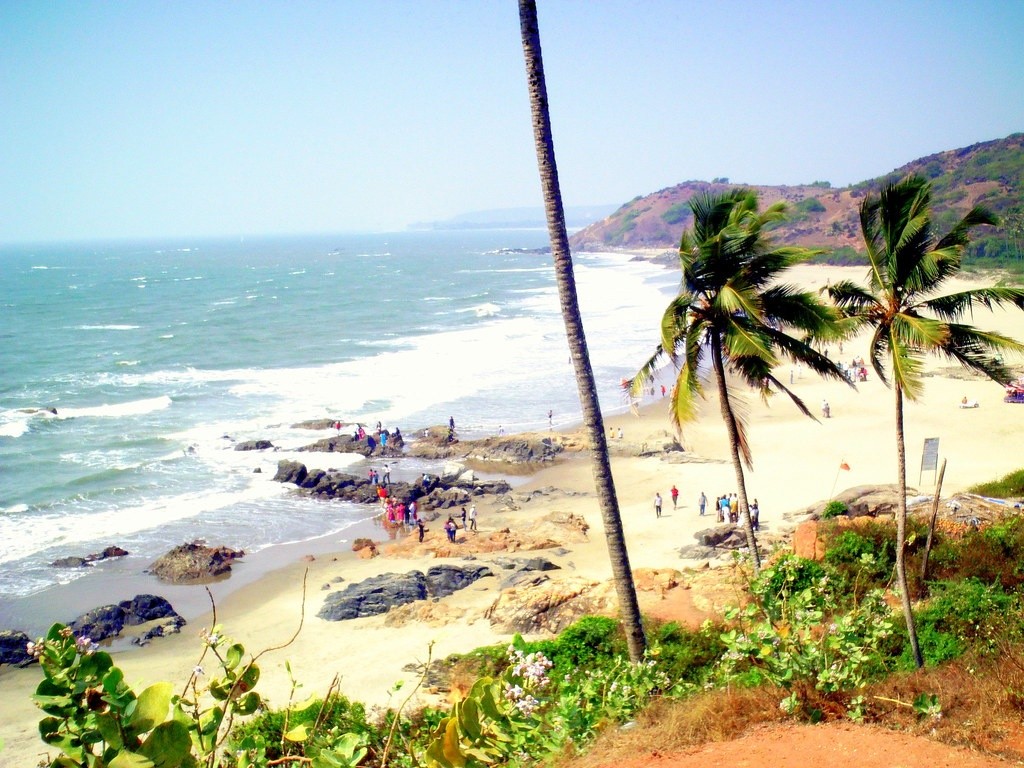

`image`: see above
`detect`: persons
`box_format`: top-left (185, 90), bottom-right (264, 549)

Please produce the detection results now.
top-left (670, 485), bottom-right (679, 510)
top-left (763, 315), bottom-right (867, 419)
top-left (653, 492), bottom-right (662, 517)
top-left (716, 493), bottom-right (760, 531)
top-left (699, 492), bottom-right (708, 515)
top-left (608, 374), bottom-right (675, 440)
top-left (961, 396), bottom-right (967, 409)
top-left (334, 408), bottom-right (554, 543)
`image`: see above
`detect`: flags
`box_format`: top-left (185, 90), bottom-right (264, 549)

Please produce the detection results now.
top-left (840, 459), bottom-right (850, 470)
